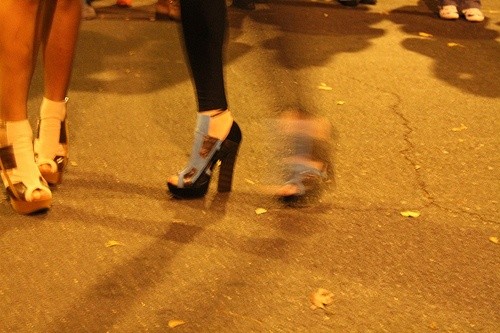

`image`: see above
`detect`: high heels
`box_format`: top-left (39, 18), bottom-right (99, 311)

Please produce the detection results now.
top-left (0, 119), bottom-right (52, 214)
top-left (33, 97), bottom-right (69, 184)
top-left (272, 114), bottom-right (338, 203)
top-left (167, 108), bottom-right (241, 199)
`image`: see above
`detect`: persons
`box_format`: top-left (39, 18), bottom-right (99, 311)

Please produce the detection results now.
top-left (0, 0), bottom-right (81, 215)
top-left (166, 0), bottom-right (340, 208)
top-left (438, 0), bottom-right (485, 22)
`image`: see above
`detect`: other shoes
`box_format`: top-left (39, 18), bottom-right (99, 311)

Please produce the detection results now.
top-left (155, 5), bottom-right (180, 22)
top-left (81, 5), bottom-right (96, 19)
top-left (461, 8), bottom-right (485, 21)
top-left (439, 6), bottom-right (459, 19)
